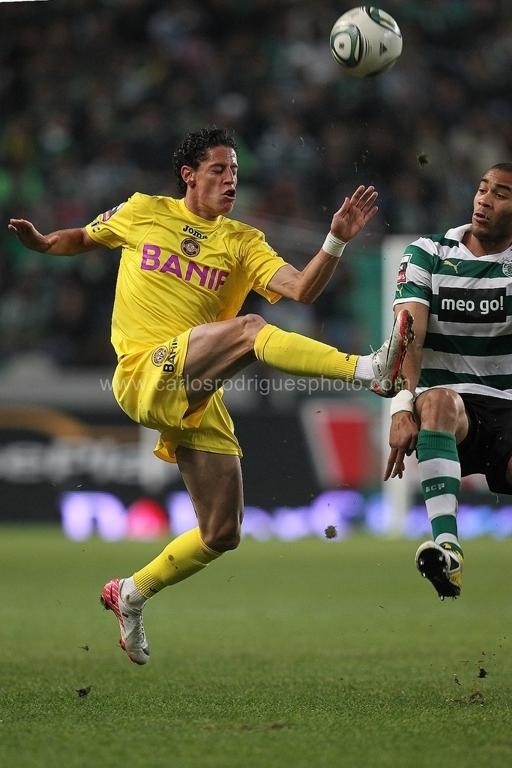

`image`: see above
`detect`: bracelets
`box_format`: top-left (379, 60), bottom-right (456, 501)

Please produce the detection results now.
top-left (316, 231), bottom-right (352, 256)
top-left (386, 387), bottom-right (416, 418)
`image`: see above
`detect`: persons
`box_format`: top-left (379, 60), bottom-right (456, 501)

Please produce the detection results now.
top-left (2, 125), bottom-right (418, 666)
top-left (383, 162), bottom-right (511, 600)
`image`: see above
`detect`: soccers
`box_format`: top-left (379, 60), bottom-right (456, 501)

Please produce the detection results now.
top-left (329, 7), bottom-right (402, 78)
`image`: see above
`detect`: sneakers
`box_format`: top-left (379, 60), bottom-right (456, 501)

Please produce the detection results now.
top-left (101, 578), bottom-right (150, 664)
top-left (369, 310), bottom-right (416, 398)
top-left (414, 540), bottom-right (464, 601)
